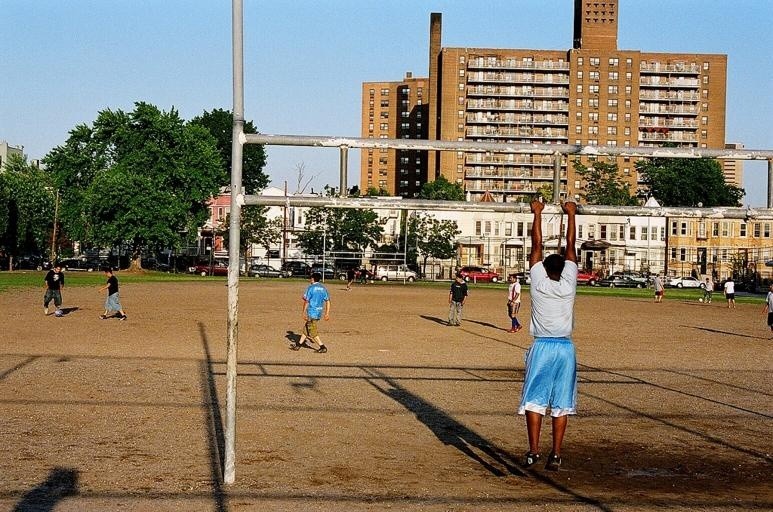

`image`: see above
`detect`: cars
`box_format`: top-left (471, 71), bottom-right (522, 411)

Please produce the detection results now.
top-left (187, 262), bottom-right (228, 277)
top-left (338, 270), bottom-right (374, 280)
top-left (508, 271), bottom-right (530, 285)
top-left (81, 257), bottom-right (166, 271)
top-left (13, 255), bottom-right (50, 272)
top-left (599, 275), bottom-right (645, 288)
top-left (55, 259), bottom-right (94, 273)
top-left (248, 265), bottom-right (284, 279)
top-left (666, 276), bottom-right (705, 289)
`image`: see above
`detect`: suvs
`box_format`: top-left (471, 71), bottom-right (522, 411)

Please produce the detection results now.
top-left (281, 261), bottom-right (311, 279)
top-left (310, 264), bottom-right (339, 279)
top-left (459, 266), bottom-right (499, 283)
top-left (576, 269), bottom-right (599, 286)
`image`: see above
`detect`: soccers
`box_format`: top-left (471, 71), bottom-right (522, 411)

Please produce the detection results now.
top-left (699, 298), bottom-right (703, 303)
top-left (55, 309), bottom-right (63, 317)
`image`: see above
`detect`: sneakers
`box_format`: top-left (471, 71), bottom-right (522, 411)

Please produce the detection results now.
top-left (521, 451), bottom-right (541, 469)
top-left (316, 348), bottom-right (326, 353)
top-left (119, 315), bottom-right (127, 321)
top-left (514, 326), bottom-right (521, 332)
top-left (290, 344), bottom-right (299, 350)
top-left (545, 454), bottom-right (561, 471)
top-left (99, 315), bottom-right (107, 319)
top-left (507, 330), bottom-right (514, 333)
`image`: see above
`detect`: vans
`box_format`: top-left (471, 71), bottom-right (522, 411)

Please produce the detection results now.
top-left (612, 271), bottom-right (647, 283)
top-left (375, 265), bottom-right (417, 283)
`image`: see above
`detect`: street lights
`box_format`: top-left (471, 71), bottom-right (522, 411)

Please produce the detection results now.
top-left (43, 185), bottom-right (59, 268)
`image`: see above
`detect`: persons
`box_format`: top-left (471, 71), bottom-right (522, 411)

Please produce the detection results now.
top-left (359, 268), bottom-right (368, 284)
top-left (98, 268), bottom-right (127, 321)
top-left (41, 265), bottom-right (64, 314)
top-left (347, 268), bottom-right (356, 290)
top-left (522, 200), bottom-right (577, 471)
top-left (654, 274), bottom-right (664, 303)
top-left (703, 278), bottom-right (714, 304)
top-left (507, 274), bottom-right (523, 333)
top-left (762, 282), bottom-right (773, 334)
top-left (292, 273), bottom-right (330, 352)
top-left (724, 277), bottom-right (736, 309)
top-left (447, 274), bottom-right (469, 326)
